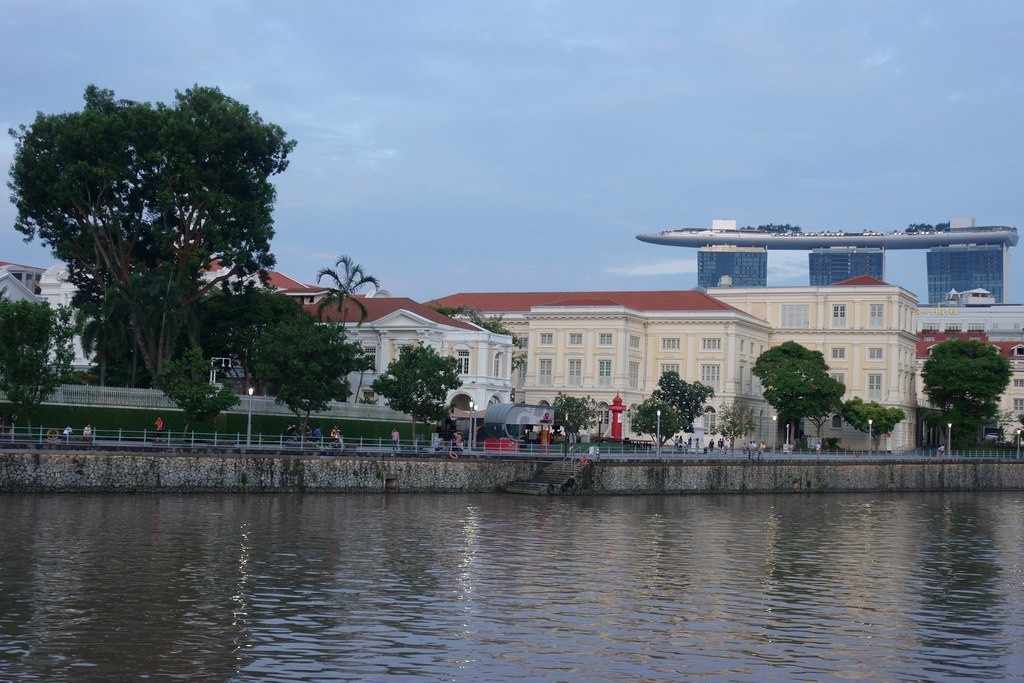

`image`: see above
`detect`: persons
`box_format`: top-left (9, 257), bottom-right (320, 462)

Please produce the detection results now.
top-left (743, 441), bottom-right (754, 459)
top-left (452, 432), bottom-right (462, 451)
top-left (937, 444), bottom-right (945, 458)
top-left (155, 417), bottom-right (163, 438)
top-left (391, 428), bottom-right (399, 450)
top-left (82, 423), bottom-right (93, 442)
top-left (758, 441), bottom-right (765, 460)
top-left (688, 437), bottom-right (692, 445)
top-left (63, 425), bottom-right (73, 439)
top-left (286, 423), bottom-right (321, 447)
top-left (328, 426), bottom-right (343, 447)
top-left (435, 444), bottom-right (442, 451)
top-left (709, 439), bottom-right (714, 451)
top-left (695, 439), bottom-right (699, 454)
top-left (718, 438), bottom-right (728, 454)
top-left (675, 435), bottom-right (683, 450)
top-left (815, 441), bottom-right (829, 457)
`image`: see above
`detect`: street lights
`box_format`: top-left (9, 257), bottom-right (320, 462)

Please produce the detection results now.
top-left (656, 409), bottom-right (661, 458)
top-left (246, 387), bottom-right (254, 446)
top-left (473, 404), bottom-right (477, 448)
top-left (466, 401), bottom-right (474, 452)
top-left (868, 419), bottom-right (873, 455)
top-left (772, 416), bottom-right (778, 450)
top-left (1016, 429), bottom-right (1022, 460)
top-left (948, 423), bottom-right (952, 456)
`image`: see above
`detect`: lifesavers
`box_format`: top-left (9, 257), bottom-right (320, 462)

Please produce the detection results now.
top-left (581, 455), bottom-right (587, 465)
top-left (449, 451), bottom-right (459, 458)
